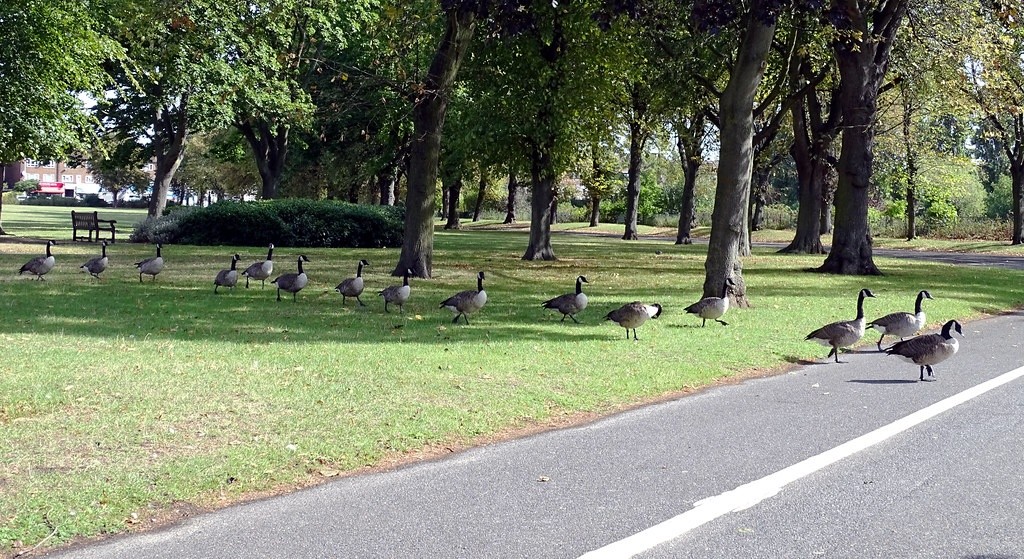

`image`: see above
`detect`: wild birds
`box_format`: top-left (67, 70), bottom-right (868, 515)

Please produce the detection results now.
top-left (439, 271), bottom-right (488, 325)
top-left (334, 259), bottom-right (370, 306)
top-left (240, 242), bottom-right (275, 291)
top-left (683, 277), bottom-right (736, 329)
top-left (270, 254), bottom-right (312, 302)
top-left (17, 239), bottom-right (59, 282)
top-left (377, 268), bottom-right (418, 313)
top-left (541, 275), bottom-right (590, 323)
top-left (602, 301), bottom-right (662, 340)
top-left (214, 253), bottom-right (242, 294)
top-left (133, 243), bottom-right (164, 285)
top-left (80, 240), bottom-right (110, 281)
top-left (804, 288), bottom-right (876, 363)
top-left (884, 319), bottom-right (966, 383)
top-left (864, 290), bottom-right (935, 354)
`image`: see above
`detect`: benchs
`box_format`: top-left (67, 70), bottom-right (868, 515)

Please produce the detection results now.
top-left (71, 210), bottom-right (117, 242)
